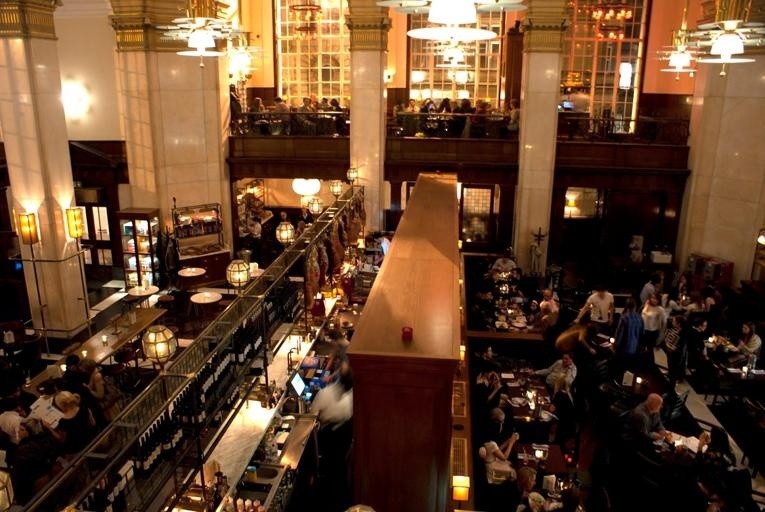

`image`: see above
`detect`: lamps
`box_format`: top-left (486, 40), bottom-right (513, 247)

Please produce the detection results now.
top-left (448, 472), bottom-right (472, 505)
top-left (155, 0), bottom-right (232, 68)
top-left (654, 0), bottom-right (765, 81)
top-left (377, 0), bottom-right (528, 43)
top-left (288, 0), bottom-right (328, 41)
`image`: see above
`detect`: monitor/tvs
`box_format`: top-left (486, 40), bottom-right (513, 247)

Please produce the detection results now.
top-left (562, 100), bottom-right (574, 108)
top-left (284, 372), bottom-right (307, 398)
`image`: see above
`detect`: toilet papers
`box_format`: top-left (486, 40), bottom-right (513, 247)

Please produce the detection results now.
top-left (127, 311), bottom-right (137, 325)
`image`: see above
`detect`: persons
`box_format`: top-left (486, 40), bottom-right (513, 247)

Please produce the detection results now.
top-left (229, 79), bottom-right (519, 138)
top-left (372, 231), bottom-right (391, 265)
top-left (471, 248), bottom-right (764, 511)
top-left (0, 352), bottom-right (127, 510)
top-left (310, 360), bottom-right (354, 444)
top-left (280, 211), bottom-right (291, 225)
top-left (321, 329), bottom-right (351, 385)
top-left (299, 207), bottom-right (314, 224)
top-left (247, 222), bottom-right (267, 267)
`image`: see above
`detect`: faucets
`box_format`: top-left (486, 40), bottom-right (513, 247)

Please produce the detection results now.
top-left (286, 347), bottom-right (295, 370)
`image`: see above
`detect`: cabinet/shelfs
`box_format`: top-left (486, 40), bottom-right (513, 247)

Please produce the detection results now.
top-left (685, 251), bottom-right (737, 289)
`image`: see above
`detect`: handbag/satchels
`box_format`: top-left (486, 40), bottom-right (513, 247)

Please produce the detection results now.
top-left (101, 384), bottom-right (119, 411)
